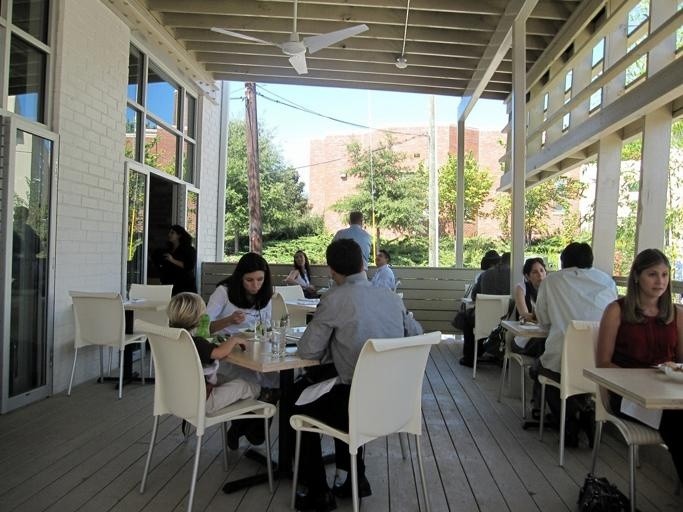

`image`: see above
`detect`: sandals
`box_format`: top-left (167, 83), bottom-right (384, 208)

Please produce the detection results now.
top-left (531, 408), bottom-right (541, 420)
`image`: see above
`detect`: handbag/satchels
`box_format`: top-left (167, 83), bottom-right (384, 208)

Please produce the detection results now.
top-left (482, 326), bottom-right (504, 357)
top-left (451, 308), bottom-right (473, 331)
top-left (577, 472), bottom-right (631, 511)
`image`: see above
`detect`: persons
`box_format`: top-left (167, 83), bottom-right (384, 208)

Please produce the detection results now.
top-left (332, 211), bottom-right (373, 272)
top-left (284, 251), bottom-right (324, 300)
top-left (285, 237), bottom-right (423, 512)
top-left (460, 251), bottom-right (511, 367)
top-left (511, 259), bottom-right (547, 360)
top-left (162, 225), bottom-right (198, 296)
top-left (207, 253), bottom-right (279, 450)
top-left (12, 206), bottom-right (41, 290)
top-left (596, 248), bottom-right (683, 499)
top-left (167, 292), bottom-right (261, 416)
top-left (371, 249), bottom-right (395, 289)
top-left (529, 242), bottom-right (619, 422)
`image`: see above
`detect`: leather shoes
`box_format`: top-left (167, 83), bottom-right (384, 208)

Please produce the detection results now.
top-left (333, 478), bottom-right (371, 500)
top-left (295, 492), bottom-right (338, 511)
top-left (460, 357), bottom-right (474, 368)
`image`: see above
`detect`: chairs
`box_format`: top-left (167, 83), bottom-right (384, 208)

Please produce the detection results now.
top-left (109, 283), bottom-right (174, 382)
top-left (264, 278), bottom-right (405, 326)
top-left (132, 317), bottom-right (279, 510)
top-left (287, 332), bottom-right (443, 511)
top-left (469, 293), bottom-right (516, 380)
top-left (67, 287), bottom-right (146, 400)
top-left (536, 320), bottom-right (600, 467)
top-left (496, 300), bottom-right (540, 419)
top-left (586, 320), bottom-right (667, 512)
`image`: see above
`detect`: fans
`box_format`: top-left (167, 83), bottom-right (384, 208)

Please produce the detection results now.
top-left (209, 1), bottom-right (369, 76)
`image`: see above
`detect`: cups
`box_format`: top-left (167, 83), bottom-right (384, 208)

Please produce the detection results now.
top-left (271, 321), bottom-right (288, 356)
top-left (253, 319), bottom-right (267, 341)
top-left (328, 279), bottom-right (334, 288)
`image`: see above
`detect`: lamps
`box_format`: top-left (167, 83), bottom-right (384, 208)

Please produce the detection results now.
top-left (392, 0), bottom-right (412, 70)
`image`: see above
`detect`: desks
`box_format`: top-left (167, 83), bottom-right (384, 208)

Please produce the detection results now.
top-left (102, 298), bottom-right (169, 391)
top-left (198, 327), bottom-right (335, 494)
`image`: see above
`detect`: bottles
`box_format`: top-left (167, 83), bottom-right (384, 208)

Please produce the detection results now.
top-left (194, 313), bottom-right (211, 338)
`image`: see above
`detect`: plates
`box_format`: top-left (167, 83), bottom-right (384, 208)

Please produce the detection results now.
top-left (658, 363), bottom-right (683, 382)
top-left (239, 328), bottom-right (255, 337)
